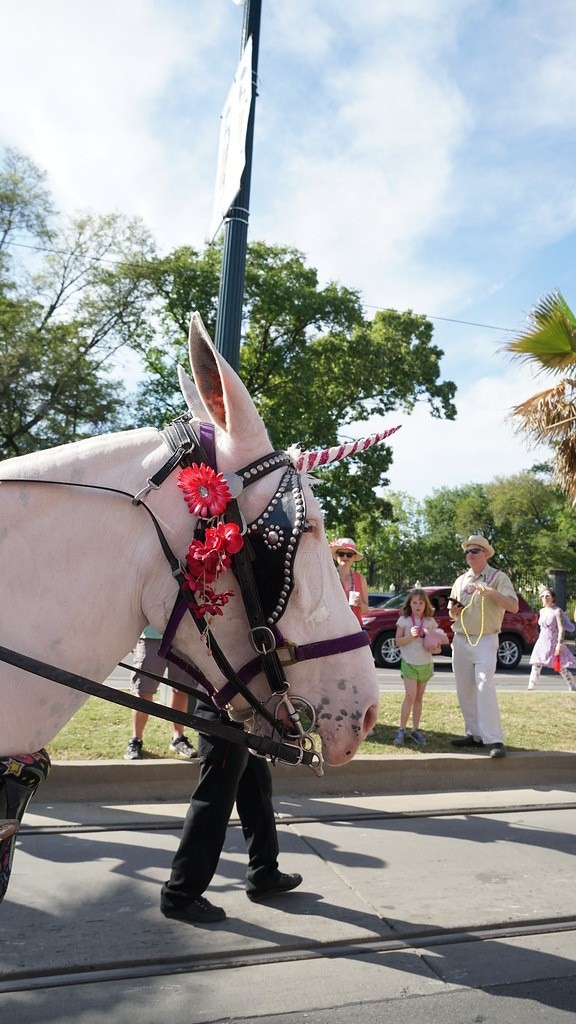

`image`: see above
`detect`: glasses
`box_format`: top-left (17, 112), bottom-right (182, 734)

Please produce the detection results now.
top-left (466, 548), bottom-right (487, 555)
top-left (540, 594), bottom-right (551, 598)
top-left (336, 551), bottom-right (355, 558)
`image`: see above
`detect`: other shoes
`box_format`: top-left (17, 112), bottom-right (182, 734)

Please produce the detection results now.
top-left (368, 729), bottom-right (373, 736)
top-left (393, 728), bottom-right (408, 745)
top-left (409, 729), bottom-right (426, 745)
top-left (451, 734), bottom-right (484, 748)
top-left (490, 743), bottom-right (506, 757)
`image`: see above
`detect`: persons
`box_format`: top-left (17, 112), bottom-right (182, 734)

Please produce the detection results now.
top-left (329, 537), bottom-right (368, 630)
top-left (446, 535), bottom-right (519, 759)
top-left (393, 590), bottom-right (441, 747)
top-left (438, 595), bottom-right (448, 613)
top-left (160, 684), bottom-right (302, 922)
top-left (528, 589), bottom-right (576, 691)
top-left (124, 624), bottom-right (201, 760)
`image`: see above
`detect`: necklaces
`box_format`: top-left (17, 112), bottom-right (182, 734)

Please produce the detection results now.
top-left (460, 585), bottom-right (485, 647)
top-left (337, 568), bottom-right (355, 609)
top-left (411, 613), bottom-right (424, 632)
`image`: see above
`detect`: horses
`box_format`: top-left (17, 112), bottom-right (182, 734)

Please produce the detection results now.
top-left (0, 311), bottom-right (379, 767)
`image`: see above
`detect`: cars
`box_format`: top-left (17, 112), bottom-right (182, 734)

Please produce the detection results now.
top-left (366, 593), bottom-right (395, 610)
top-left (359, 586), bottom-right (541, 670)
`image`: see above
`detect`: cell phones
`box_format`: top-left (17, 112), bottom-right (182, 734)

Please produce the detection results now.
top-left (448, 597), bottom-right (466, 610)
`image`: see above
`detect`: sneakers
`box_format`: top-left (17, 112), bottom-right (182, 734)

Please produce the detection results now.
top-left (159, 897), bottom-right (226, 922)
top-left (123, 738), bottom-right (143, 759)
top-left (170, 736), bottom-right (198, 758)
top-left (248, 874), bottom-right (303, 902)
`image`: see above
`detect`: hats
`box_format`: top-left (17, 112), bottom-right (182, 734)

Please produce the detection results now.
top-left (330, 538), bottom-right (364, 561)
top-left (423, 627), bottom-right (449, 650)
top-left (461, 535), bottom-right (495, 559)
